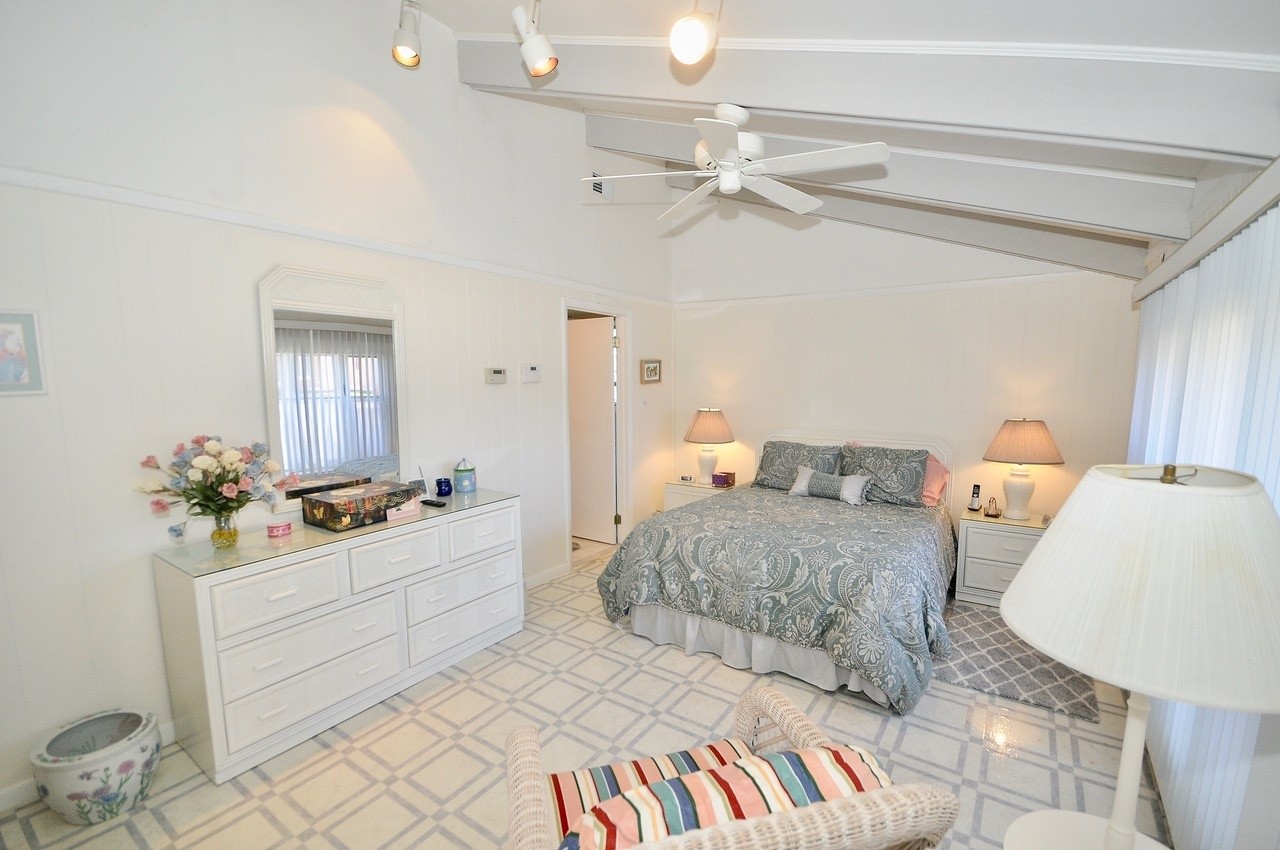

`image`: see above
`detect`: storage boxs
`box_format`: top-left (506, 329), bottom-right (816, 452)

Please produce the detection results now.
top-left (285, 471), bottom-right (371, 502)
top-left (302, 480), bottom-right (419, 533)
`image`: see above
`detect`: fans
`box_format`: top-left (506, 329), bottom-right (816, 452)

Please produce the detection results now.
top-left (575, 101), bottom-right (889, 215)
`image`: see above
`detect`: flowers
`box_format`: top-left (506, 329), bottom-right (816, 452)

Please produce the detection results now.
top-left (141, 436), bottom-right (299, 534)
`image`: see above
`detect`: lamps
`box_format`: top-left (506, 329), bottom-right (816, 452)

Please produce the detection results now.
top-left (512, 0), bottom-right (559, 78)
top-left (1001, 463), bottom-right (1280, 850)
top-left (669, 0), bottom-right (723, 65)
top-left (683, 408), bottom-right (735, 484)
top-left (983, 418), bottom-right (1064, 520)
top-left (392, 0), bottom-right (422, 68)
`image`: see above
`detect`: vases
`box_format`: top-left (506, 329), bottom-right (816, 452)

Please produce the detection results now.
top-left (28, 707), bottom-right (163, 826)
top-left (210, 517), bottom-right (239, 549)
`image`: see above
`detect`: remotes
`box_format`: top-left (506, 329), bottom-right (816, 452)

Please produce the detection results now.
top-left (420, 499), bottom-right (446, 507)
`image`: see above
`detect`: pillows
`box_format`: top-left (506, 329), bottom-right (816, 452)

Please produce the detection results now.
top-left (750, 441), bottom-right (951, 508)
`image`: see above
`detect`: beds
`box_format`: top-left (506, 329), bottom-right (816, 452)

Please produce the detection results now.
top-left (595, 435), bottom-right (960, 715)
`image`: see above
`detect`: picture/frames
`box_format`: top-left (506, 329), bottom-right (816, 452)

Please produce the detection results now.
top-left (0, 308), bottom-right (48, 397)
top-left (640, 359), bottom-right (662, 384)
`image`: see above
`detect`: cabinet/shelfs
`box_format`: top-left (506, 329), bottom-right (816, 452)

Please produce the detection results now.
top-left (148, 489), bottom-right (522, 789)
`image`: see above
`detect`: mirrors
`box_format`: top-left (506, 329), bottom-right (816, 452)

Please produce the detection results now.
top-left (258, 266), bottom-right (410, 512)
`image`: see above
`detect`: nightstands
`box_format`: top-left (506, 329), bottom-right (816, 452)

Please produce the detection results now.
top-left (955, 506), bottom-right (1053, 608)
top-left (664, 481), bottom-right (738, 513)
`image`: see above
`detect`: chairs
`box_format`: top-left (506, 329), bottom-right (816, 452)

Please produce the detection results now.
top-left (510, 688), bottom-right (960, 850)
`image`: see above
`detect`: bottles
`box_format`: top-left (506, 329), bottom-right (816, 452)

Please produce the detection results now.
top-left (31, 708), bottom-right (161, 824)
top-left (453, 458), bottom-right (477, 493)
top-left (434, 478), bottom-right (453, 497)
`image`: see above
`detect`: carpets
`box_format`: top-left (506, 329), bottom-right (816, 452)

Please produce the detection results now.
top-left (932, 600), bottom-right (1100, 723)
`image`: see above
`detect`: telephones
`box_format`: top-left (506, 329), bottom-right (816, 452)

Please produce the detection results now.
top-left (967, 484), bottom-right (983, 511)
